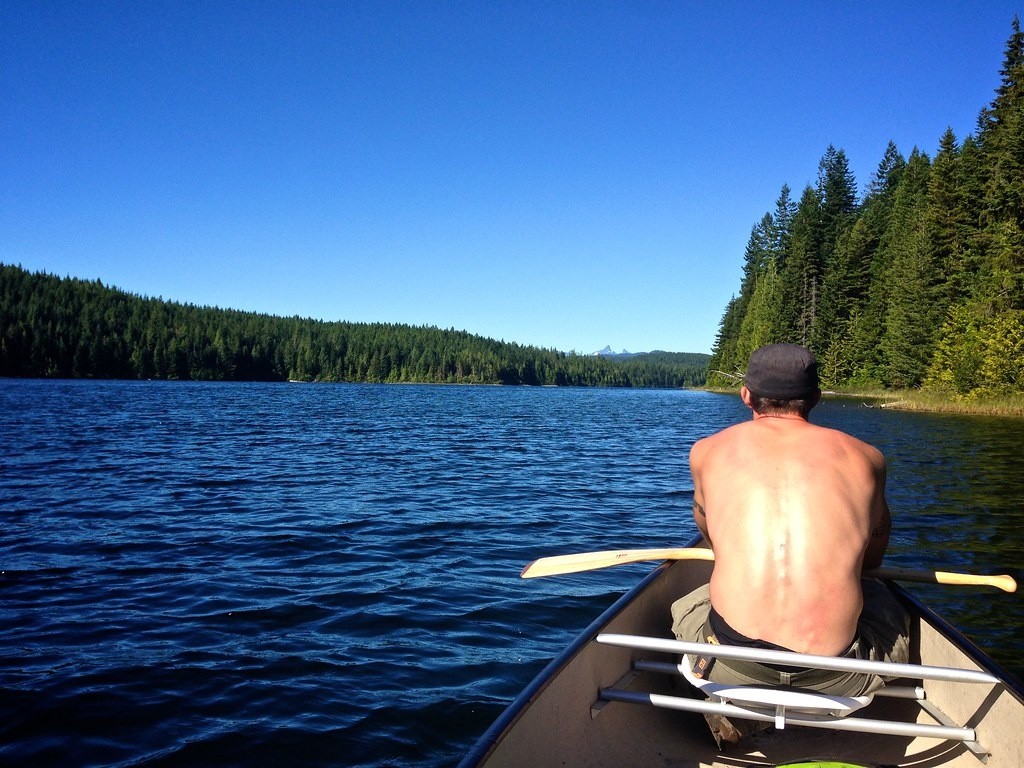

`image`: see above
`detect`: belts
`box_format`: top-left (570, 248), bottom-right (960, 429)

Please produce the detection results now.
top-left (702, 616), bottom-right (862, 687)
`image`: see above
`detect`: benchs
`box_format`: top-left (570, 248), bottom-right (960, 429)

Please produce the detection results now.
top-left (591, 634), bottom-right (1003, 760)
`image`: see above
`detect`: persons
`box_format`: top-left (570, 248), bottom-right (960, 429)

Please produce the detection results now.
top-left (670, 344), bottom-right (911, 750)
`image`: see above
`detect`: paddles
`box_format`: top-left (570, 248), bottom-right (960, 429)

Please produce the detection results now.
top-left (519, 546), bottom-right (1019, 594)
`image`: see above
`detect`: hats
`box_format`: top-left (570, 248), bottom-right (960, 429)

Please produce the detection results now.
top-left (745, 344), bottom-right (820, 399)
